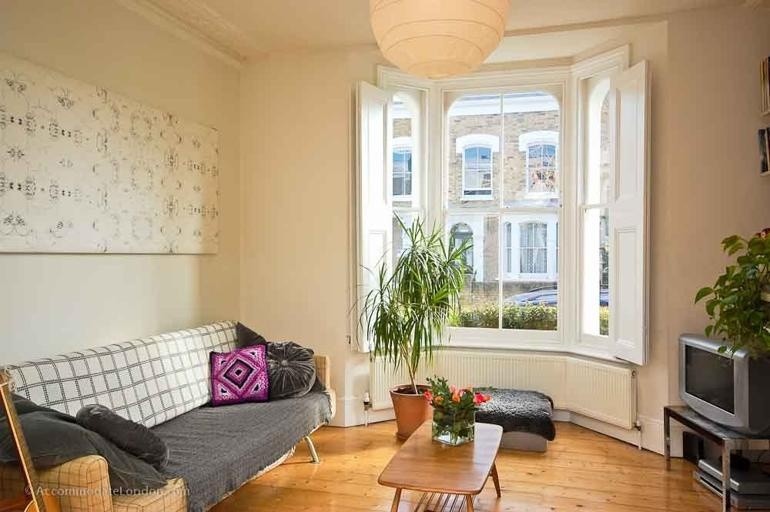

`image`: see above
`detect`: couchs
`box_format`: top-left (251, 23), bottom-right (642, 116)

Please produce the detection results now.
top-left (0, 318), bottom-right (337, 512)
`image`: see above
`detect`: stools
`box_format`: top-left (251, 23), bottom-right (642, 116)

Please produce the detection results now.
top-left (472, 388), bottom-right (556, 452)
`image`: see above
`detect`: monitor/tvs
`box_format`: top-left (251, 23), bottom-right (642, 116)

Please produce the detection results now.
top-left (679, 333), bottom-right (770, 436)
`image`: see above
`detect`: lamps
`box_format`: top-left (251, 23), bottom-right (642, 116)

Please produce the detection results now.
top-left (368, 1), bottom-right (507, 79)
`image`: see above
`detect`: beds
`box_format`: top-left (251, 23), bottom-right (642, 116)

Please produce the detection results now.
top-left (376, 418), bottom-right (503, 511)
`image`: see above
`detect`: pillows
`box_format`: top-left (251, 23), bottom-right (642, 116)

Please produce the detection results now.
top-left (2, 394), bottom-right (167, 495)
top-left (210, 321), bottom-right (316, 405)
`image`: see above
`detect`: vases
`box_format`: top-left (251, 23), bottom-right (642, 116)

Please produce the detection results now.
top-left (432, 408), bottom-right (476, 446)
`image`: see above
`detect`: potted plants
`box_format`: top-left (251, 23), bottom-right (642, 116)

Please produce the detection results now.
top-left (347, 212), bottom-right (474, 441)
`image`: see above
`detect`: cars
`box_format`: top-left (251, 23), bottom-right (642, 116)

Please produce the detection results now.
top-left (505, 285), bottom-right (608, 306)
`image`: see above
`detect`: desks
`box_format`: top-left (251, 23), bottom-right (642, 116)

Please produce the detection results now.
top-left (663, 404), bottom-right (770, 511)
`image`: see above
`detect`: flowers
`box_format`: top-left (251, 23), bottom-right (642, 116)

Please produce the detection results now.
top-left (423, 373), bottom-right (491, 440)
